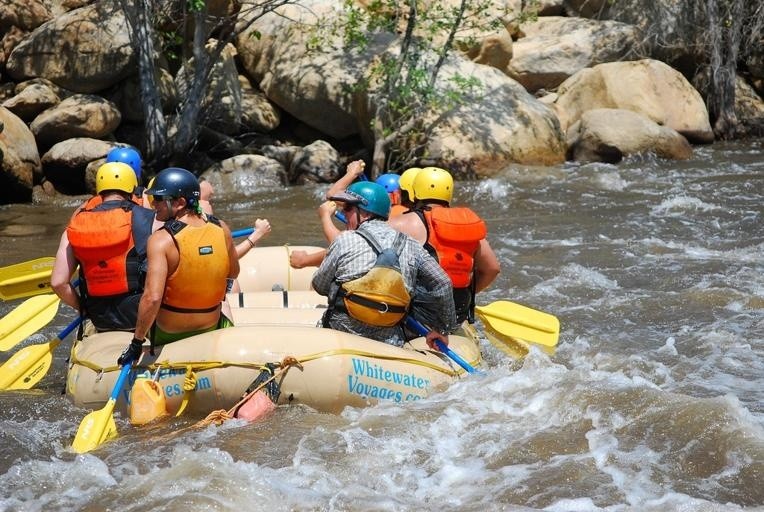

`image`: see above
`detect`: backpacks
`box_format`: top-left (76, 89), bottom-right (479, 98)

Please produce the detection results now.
top-left (342, 228), bottom-right (411, 327)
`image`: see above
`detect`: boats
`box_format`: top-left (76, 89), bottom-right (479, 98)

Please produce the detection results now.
top-left (66, 245), bottom-right (490, 413)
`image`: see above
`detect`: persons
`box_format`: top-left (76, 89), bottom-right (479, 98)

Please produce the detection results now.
top-left (47, 146), bottom-right (271, 369)
top-left (285, 160), bottom-right (502, 356)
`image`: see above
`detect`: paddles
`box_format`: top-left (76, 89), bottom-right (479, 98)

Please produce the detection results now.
top-left (484, 319), bottom-right (556, 359)
top-left (0, 276), bottom-right (81, 351)
top-left (71, 362), bottom-right (132, 454)
top-left (0, 310), bottom-right (87, 391)
top-left (1, 227), bottom-right (256, 302)
top-left (474, 302), bottom-right (561, 347)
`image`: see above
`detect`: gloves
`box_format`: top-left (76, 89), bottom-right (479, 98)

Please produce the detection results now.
top-left (118, 335), bottom-right (146, 367)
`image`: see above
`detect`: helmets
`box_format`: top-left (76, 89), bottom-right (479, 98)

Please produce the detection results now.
top-left (327, 181), bottom-right (389, 219)
top-left (146, 167), bottom-right (201, 200)
top-left (96, 147), bottom-right (144, 195)
top-left (375, 167), bottom-right (454, 205)
